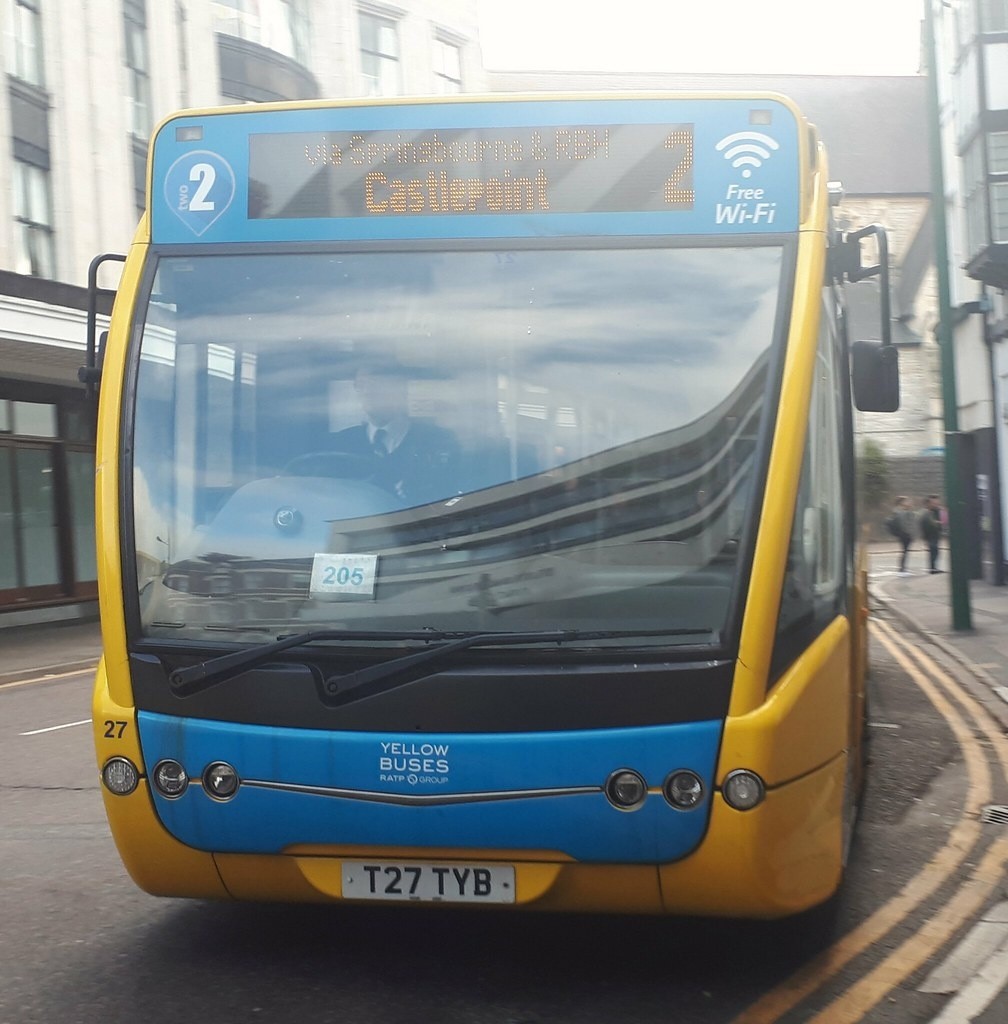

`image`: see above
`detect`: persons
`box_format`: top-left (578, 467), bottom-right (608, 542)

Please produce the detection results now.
top-left (891, 495), bottom-right (914, 571)
top-left (305, 354), bottom-right (460, 506)
top-left (920, 495), bottom-right (945, 575)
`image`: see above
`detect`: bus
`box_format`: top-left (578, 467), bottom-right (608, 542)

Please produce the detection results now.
top-left (78, 87), bottom-right (903, 927)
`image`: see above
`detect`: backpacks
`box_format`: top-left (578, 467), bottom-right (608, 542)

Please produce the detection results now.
top-left (884, 513), bottom-right (901, 538)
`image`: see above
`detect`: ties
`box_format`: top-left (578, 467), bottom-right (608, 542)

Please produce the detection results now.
top-left (372, 429), bottom-right (389, 455)
